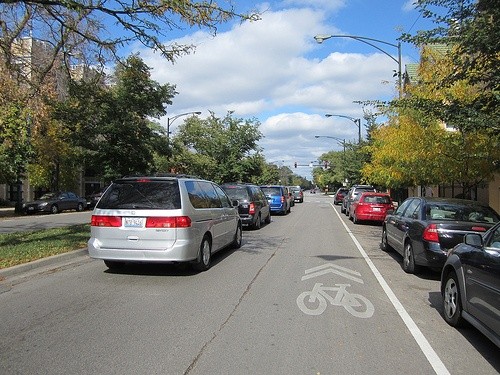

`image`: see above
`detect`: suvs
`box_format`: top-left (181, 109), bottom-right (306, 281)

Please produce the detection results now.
top-left (341, 184), bottom-right (377, 216)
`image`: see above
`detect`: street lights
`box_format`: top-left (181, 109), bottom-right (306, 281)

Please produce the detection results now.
top-left (314, 33), bottom-right (402, 107)
top-left (166, 112), bottom-right (201, 162)
top-left (315, 135), bottom-right (346, 159)
top-left (325, 114), bottom-right (362, 144)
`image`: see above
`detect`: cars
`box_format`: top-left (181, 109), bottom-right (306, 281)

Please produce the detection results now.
top-left (440, 219), bottom-right (500, 353)
top-left (348, 191), bottom-right (394, 224)
top-left (25, 191), bottom-right (87, 215)
top-left (334, 188), bottom-right (350, 205)
top-left (382, 196), bottom-right (500, 273)
top-left (82, 188), bottom-right (107, 209)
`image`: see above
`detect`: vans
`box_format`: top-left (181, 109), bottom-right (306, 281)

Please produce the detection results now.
top-left (87, 173), bottom-right (243, 271)
top-left (260, 185), bottom-right (295, 216)
top-left (220, 182), bottom-right (271, 229)
top-left (285, 185), bottom-right (305, 203)
